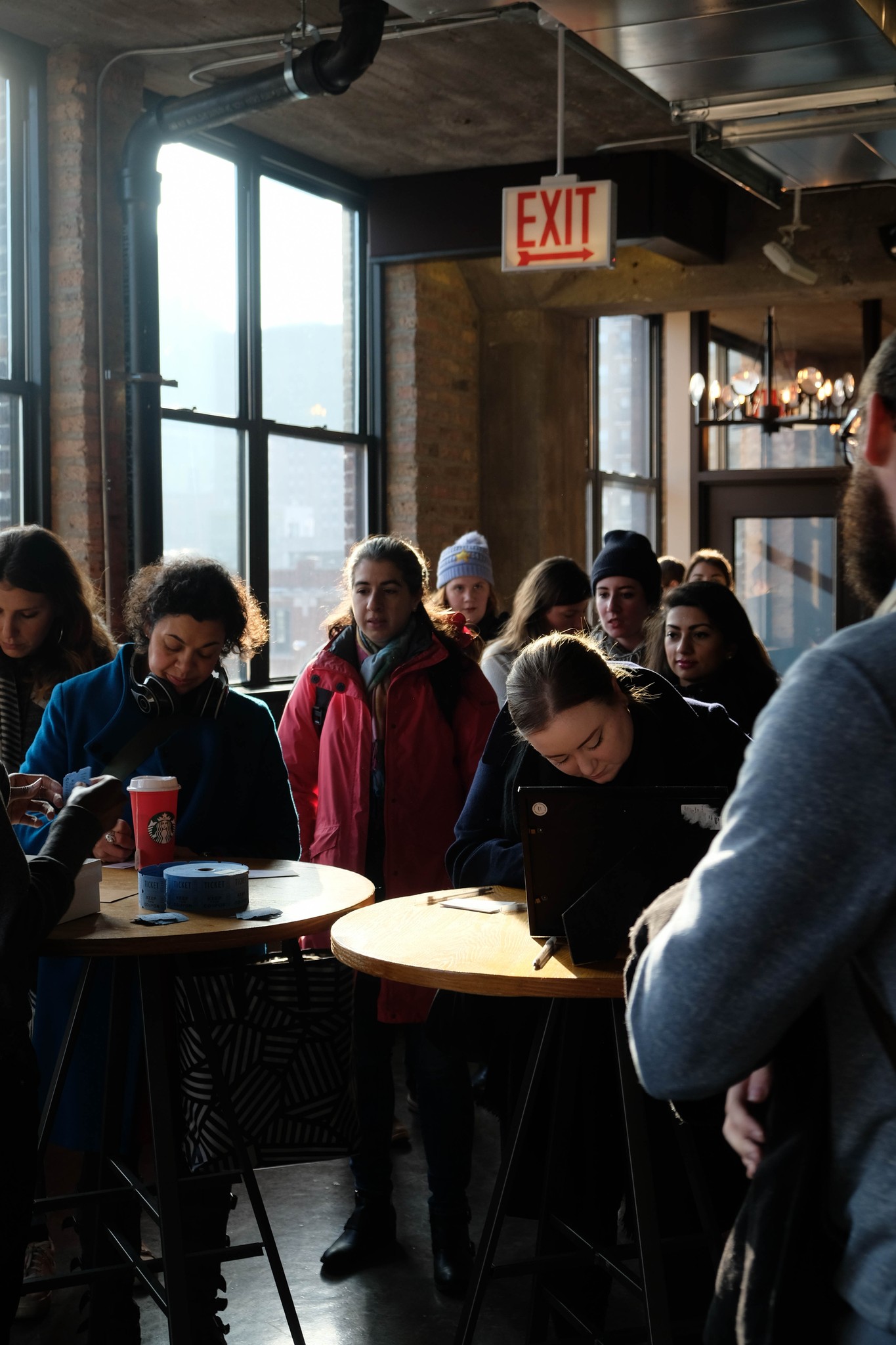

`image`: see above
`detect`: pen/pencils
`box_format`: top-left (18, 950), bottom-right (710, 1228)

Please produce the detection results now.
top-left (426, 885), bottom-right (494, 905)
top-left (533, 935), bottom-right (558, 971)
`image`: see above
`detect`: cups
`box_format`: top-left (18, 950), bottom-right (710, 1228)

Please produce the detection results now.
top-left (126, 773), bottom-right (181, 872)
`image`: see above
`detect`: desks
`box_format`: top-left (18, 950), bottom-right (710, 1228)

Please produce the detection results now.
top-left (330, 879), bottom-right (701, 1343)
top-left (1, 856), bottom-right (372, 1343)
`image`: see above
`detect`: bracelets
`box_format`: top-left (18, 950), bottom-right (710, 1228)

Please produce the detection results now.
top-left (199, 850), bottom-right (212, 857)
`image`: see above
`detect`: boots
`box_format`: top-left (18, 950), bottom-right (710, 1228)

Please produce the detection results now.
top-left (320, 1186), bottom-right (397, 1276)
top-left (430, 1206), bottom-right (476, 1296)
top-left (178, 1190), bottom-right (238, 1345)
top-left (61, 1180), bottom-right (141, 1345)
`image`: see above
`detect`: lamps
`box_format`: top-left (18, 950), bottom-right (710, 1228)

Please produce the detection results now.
top-left (686, 300), bottom-right (857, 438)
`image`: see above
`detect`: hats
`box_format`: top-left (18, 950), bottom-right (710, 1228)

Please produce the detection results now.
top-left (589, 530), bottom-right (662, 605)
top-left (436, 531), bottom-right (495, 591)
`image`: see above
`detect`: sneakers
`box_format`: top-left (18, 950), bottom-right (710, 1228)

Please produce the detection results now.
top-left (14, 1237), bottom-right (56, 1318)
top-left (133, 1239), bottom-right (159, 1286)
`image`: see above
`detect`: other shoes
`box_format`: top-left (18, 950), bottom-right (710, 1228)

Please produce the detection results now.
top-left (392, 1115), bottom-right (411, 1146)
top-left (406, 1094), bottom-right (419, 1113)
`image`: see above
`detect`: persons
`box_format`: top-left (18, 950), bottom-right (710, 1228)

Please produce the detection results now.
top-left (14, 555), bottom-right (303, 1345)
top-left (1, 521), bottom-right (159, 1317)
top-left (280, 524), bottom-right (780, 1298)
top-left (1, 761), bottom-right (125, 1345)
top-left (618, 334), bottom-right (896, 1345)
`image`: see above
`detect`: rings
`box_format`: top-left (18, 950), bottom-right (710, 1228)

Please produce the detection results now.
top-left (105, 830), bottom-right (116, 846)
